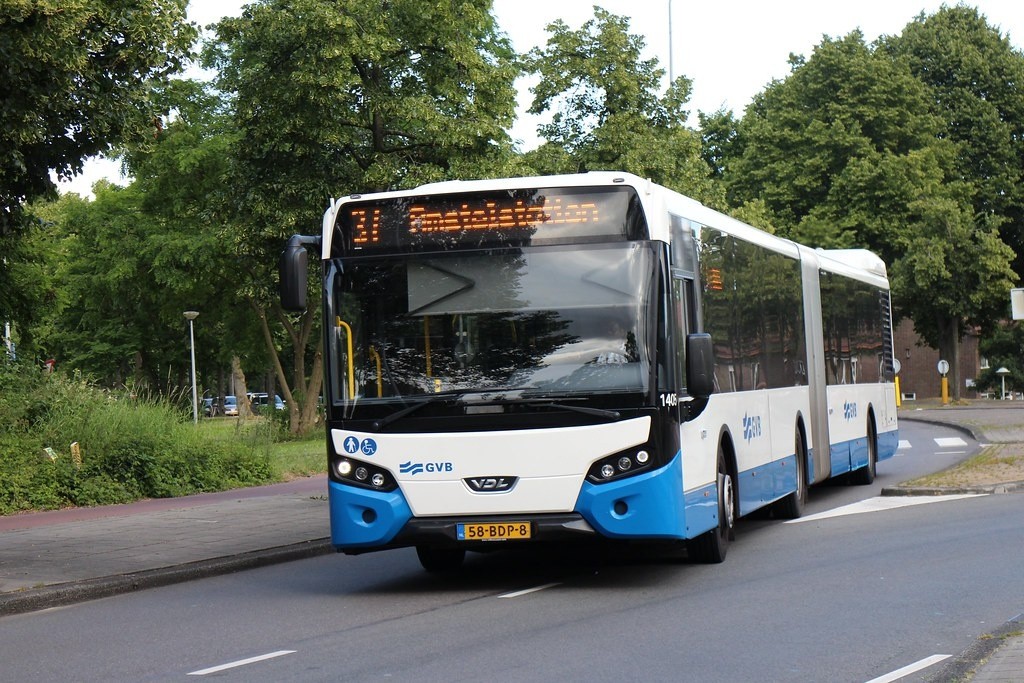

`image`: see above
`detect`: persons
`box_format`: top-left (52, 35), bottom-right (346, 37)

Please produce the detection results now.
top-left (577, 314), bottom-right (636, 367)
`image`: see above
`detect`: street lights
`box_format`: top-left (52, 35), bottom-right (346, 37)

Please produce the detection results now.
top-left (183, 311), bottom-right (201, 426)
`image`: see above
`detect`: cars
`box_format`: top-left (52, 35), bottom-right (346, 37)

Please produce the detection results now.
top-left (245, 392), bottom-right (325, 418)
top-left (981, 392), bottom-right (1016, 401)
top-left (211, 395), bottom-right (245, 416)
top-left (203, 399), bottom-right (214, 417)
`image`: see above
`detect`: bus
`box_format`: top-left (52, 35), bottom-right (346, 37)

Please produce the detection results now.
top-left (281, 173), bottom-right (899, 568)
